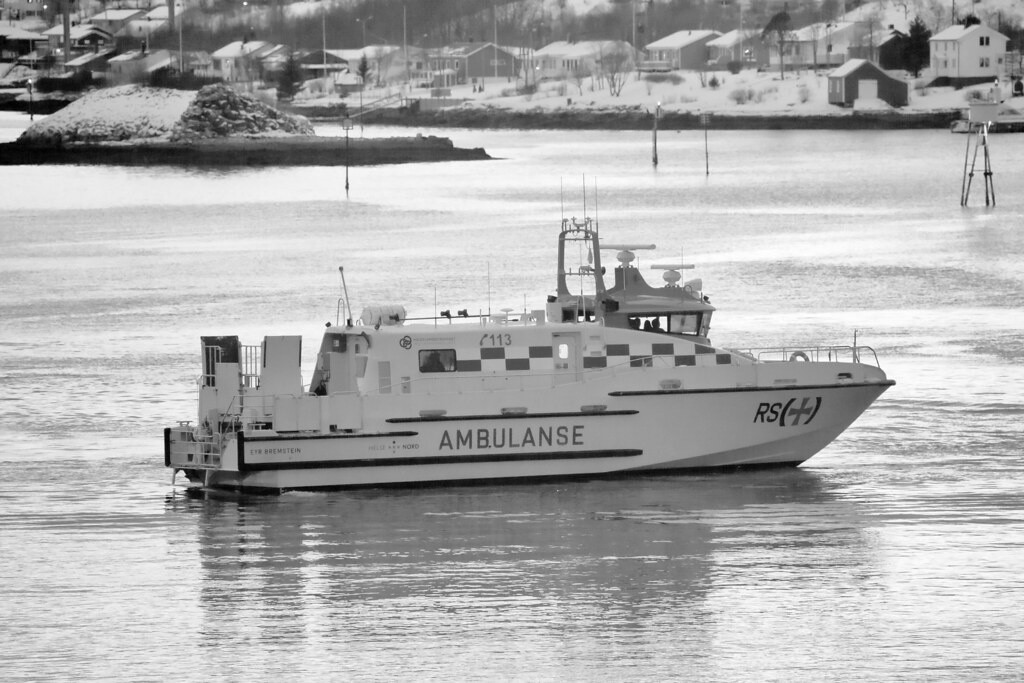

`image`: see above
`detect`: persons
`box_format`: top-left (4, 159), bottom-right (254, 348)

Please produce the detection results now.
top-left (630, 317), bottom-right (665, 333)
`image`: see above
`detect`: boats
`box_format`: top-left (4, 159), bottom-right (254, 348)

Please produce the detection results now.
top-left (163, 174), bottom-right (897, 492)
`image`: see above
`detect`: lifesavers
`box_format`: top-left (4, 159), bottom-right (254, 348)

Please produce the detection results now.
top-left (791, 351), bottom-right (810, 362)
